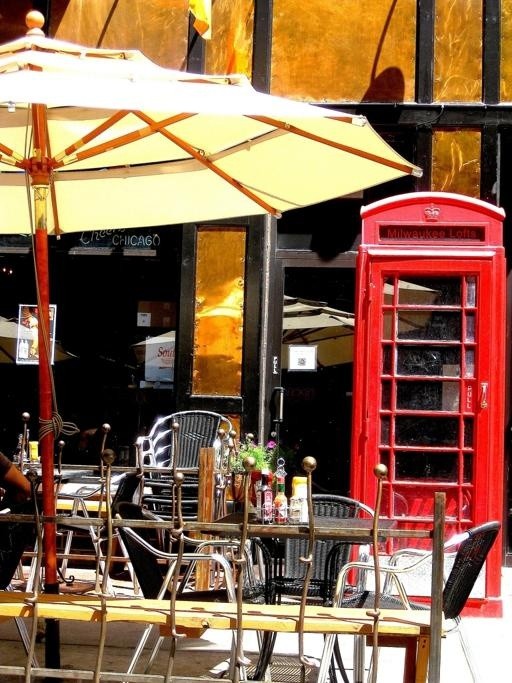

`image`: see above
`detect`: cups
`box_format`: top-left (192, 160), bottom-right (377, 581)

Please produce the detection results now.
top-left (28, 439), bottom-right (39, 462)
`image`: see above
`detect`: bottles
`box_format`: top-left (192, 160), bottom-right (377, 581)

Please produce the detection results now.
top-left (16, 434), bottom-right (23, 462)
top-left (255, 467), bottom-right (309, 525)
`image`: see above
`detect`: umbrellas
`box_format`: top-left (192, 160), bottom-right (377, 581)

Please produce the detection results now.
top-left (382, 279), bottom-right (442, 337)
top-left (0, 11), bottom-right (426, 671)
top-left (282, 292), bottom-right (357, 374)
top-left (0, 315), bottom-right (79, 364)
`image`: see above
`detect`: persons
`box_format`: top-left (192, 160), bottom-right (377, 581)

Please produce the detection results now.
top-left (0, 450), bottom-right (96, 593)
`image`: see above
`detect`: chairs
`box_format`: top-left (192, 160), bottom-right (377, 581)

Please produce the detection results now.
top-left (0, 403), bottom-right (505, 682)
